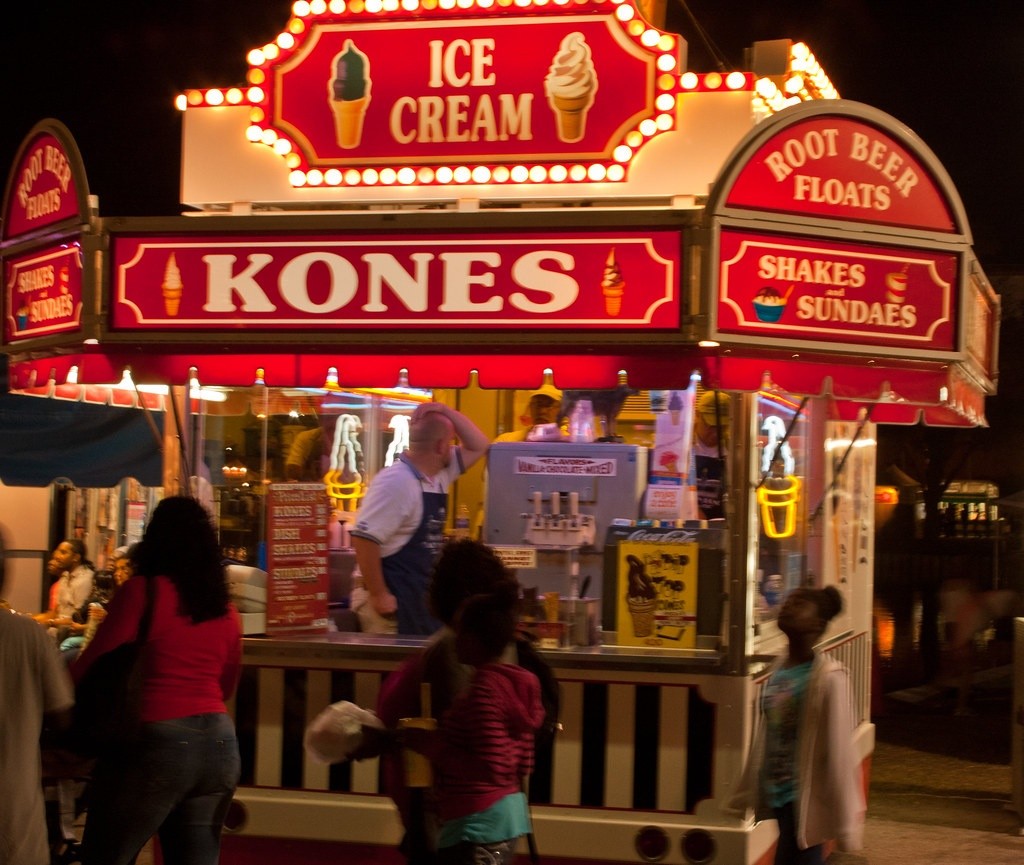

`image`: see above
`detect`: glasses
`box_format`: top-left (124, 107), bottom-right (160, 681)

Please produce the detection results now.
top-left (529, 398), bottom-right (560, 409)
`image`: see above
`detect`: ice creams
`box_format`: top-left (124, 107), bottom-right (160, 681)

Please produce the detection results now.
top-left (668, 390), bottom-right (684, 424)
top-left (329, 37), bottom-right (375, 148)
top-left (603, 248), bottom-right (623, 316)
top-left (547, 31), bottom-right (598, 142)
top-left (761, 415), bottom-right (800, 538)
top-left (324, 414), bottom-right (365, 515)
top-left (161, 251), bottom-right (182, 316)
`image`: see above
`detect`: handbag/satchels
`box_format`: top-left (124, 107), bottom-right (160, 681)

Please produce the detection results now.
top-left (66, 575), bottom-right (156, 755)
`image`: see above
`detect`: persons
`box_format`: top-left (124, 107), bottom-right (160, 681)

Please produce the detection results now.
top-left (722, 585), bottom-right (864, 865)
top-left (0, 521), bottom-right (77, 865)
top-left (493, 384), bottom-right (570, 443)
top-left (349, 402), bottom-right (489, 638)
top-left (69, 495), bottom-right (244, 865)
top-left (32, 538), bottom-right (142, 665)
top-left (340, 541), bottom-right (560, 865)
top-left (281, 407), bottom-right (347, 484)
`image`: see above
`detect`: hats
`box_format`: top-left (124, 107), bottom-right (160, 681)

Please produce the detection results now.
top-left (529, 385), bottom-right (563, 401)
